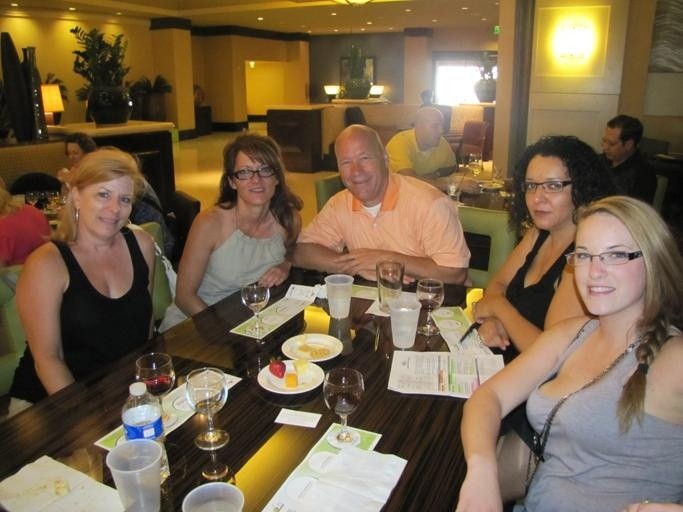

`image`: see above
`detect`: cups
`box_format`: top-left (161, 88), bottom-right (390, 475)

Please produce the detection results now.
top-left (27, 191), bottom-right (59, 205)
top-left (468, 152), bottom-right (482, 177)
top-left (325, 259), bottom-right (421, 348)
top-left (106, 440), bottom-right (245, 512)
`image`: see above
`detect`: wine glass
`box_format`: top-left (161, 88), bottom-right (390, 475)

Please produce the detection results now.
top-left (416, 278), bottom-right (444, 336)
top-left (242, 279), bottom-right (271, 337)
top-left (135, 353), bottom-right (227, 450)
top-left (324, 368), bottom-right (364, 443)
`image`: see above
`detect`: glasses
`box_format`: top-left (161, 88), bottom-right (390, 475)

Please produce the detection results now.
top-left (563, 250), bottom-right (642, 267)
top-left (520, 180), bottom-right (573, 192)
top-left (231, 165), bottom-right (276, 180)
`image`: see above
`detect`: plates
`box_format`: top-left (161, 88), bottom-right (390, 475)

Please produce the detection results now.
top-left (257, 333), bottom-right (343, 394)
top-left (480, 183), bottom-right (502, 190)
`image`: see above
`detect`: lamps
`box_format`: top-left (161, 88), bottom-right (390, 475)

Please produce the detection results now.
top-left (323, 85), bottom-right (340, 102)
top-left (369, 85), bottom-right (384, 98)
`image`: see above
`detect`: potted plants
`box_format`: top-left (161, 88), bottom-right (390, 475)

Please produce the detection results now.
top-left (463, 27), bottom-right (495, 102)
top-left (343, 0), bottom-right (382, 99)
top-left (131, 74), bottom-right (173, 120)
top-left (70, 26), bottom-right (134, 125)
top-left (41, 72), bottom-right (69, 125)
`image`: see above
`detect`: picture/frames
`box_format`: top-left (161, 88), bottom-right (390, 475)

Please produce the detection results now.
top-left (339, 56), bottom-right (376, 98)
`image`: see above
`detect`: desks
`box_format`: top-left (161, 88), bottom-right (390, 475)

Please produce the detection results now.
top-left (46, 119), bottom-right (176, 193)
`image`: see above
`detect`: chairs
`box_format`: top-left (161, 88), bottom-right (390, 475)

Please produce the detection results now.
top-left (1, 223), bottom-right (174, 321)
top-left (165, 190), bottom-right (201, 272)
top-left (443, 104), bottom-right (484, 152)
top-left (314, 175), bottom-right (345, 213)
top-left (2, 276), bottom-right (28, 396)
top-left (460, 118), bottom-right (493, 159)
top-left (10, 172), bottom-right (61, 194)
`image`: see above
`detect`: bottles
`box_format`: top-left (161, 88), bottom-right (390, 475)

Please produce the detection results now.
top-left (122, 382), bottom-right (170, 484)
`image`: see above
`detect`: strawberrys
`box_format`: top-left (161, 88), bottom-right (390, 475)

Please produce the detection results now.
top-left (269, 357), bottom-right (286, 378)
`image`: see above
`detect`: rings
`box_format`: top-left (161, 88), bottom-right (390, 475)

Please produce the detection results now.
top-left (642, 500), bottom-right (649, 504)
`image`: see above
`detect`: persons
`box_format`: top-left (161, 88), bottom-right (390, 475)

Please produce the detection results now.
top-left (470, 135), bottom-right (611, 430)
top-left (6, 146), bottom-right (157, 421)
top-left (455, 194), bottom-right (683, 512)
top-left (150, 132), bottom-right (305, 337)
top-left (595, 114), bottom-right (658, 207)
top-left (383, 106), bottom-right (459, 183)
top-left (290, 124), bottom-right (474, 286)
top-left (0, 133), bottom-right (176, 269)
